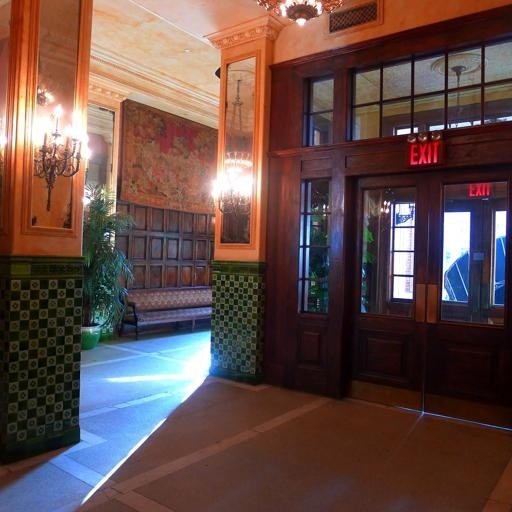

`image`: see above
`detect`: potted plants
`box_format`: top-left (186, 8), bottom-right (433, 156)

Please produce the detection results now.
top-left (81, 183), bottom-right (137, 350)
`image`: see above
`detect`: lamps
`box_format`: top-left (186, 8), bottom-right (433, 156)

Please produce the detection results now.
top-left (37, 59), bottom-right (61, 111)
top-left (224, 76), bottom-right (256, 174)
top-left (258, 0), bottom-right (345, 26)
top-left (33, 104), bottom-right (91, 213)
top-left (210, 150), bottom-right (253, 219)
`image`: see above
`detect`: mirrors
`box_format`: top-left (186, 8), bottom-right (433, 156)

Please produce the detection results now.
top-left (21, 1), bottom-right (87, 238)
top-left (216, 48), bottom-right (261, 248)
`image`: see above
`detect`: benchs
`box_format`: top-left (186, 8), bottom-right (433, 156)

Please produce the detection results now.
top-left (125, 287), bottom-right (212, 340)
